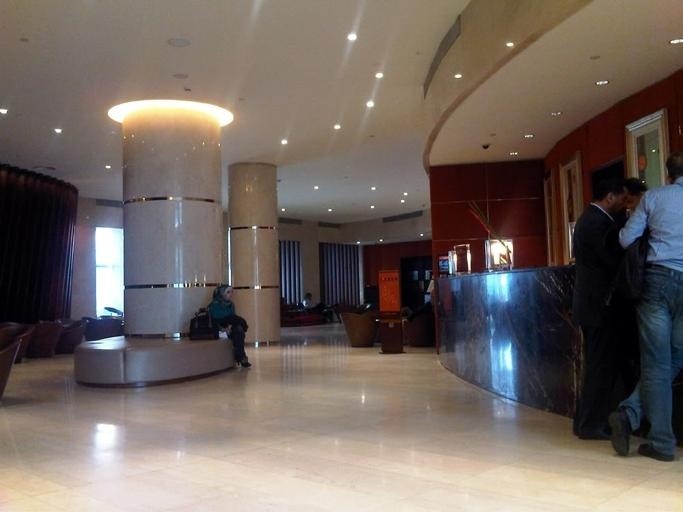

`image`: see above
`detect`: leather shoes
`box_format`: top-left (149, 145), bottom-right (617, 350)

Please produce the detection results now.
top-left (608, 408), bottom-right (631, 456)
top-left (638, 443), bottom-right (674, 462)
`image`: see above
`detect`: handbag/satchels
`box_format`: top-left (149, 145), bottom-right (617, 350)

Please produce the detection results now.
top-left (188, 308), bottom-right (220, 340)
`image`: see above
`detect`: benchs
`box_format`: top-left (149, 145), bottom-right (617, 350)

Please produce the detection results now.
top-left (75, 335), bottom-right (235, 389)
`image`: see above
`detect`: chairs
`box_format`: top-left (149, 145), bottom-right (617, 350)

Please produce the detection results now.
top-left (0, 305), bottom-right (124, 399)
top-left (280, 299), bottom-right (435, 355)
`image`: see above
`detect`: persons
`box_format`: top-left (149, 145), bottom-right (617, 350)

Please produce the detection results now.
top-left (208, 285), bottom-right (252, 367)
top-left (303, 293), bottom-right (313, 308)
top-left (572, 166), bottom-right (641, 440)
top-left (623, 177), bottom-right (647, 214)
top-left (608, 152), bottom-right (683, 461)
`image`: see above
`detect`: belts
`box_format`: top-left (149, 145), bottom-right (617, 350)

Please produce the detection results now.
top-left (644, 263), bottom-right (683, 277)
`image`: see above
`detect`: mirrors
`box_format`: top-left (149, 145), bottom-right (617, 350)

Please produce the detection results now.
top-left (622, 106), bottom-right (671, 192)
top-left (557, 150), bottom-right (585, 268)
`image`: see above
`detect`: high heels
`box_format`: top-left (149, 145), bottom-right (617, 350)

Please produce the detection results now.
top-left (234, 354), bottom-right (252, 367)
